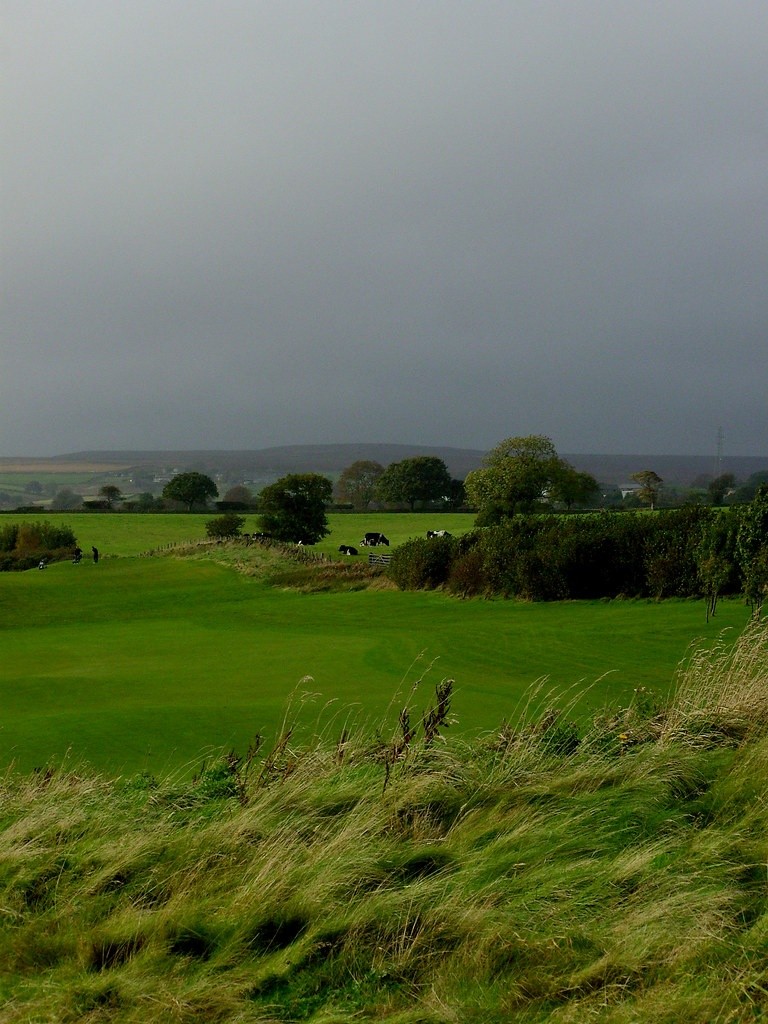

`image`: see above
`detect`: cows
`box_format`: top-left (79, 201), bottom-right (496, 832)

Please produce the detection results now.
top-left (337, 545), bottom-right (358, 555)
top-left (427, 530), bottom-right (452, 540)
top-left (359, 533), bottom-right (389, 548)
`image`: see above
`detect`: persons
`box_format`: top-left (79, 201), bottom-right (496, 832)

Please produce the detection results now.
top-left (91, 545), bottom-right (99, 563)
top-left (74, 546), bottom-right (82, 561)
top-left (296, 540), bottom-right (304, 548)
